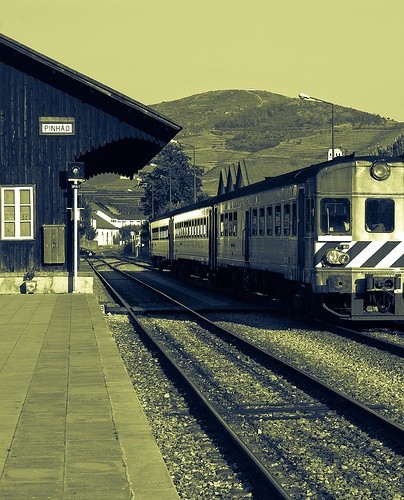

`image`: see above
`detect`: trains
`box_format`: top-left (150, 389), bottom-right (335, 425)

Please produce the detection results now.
top-left (147, 152), bottom-right (404, 321)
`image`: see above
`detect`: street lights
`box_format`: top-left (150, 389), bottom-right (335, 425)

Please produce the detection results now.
top-left (298, 93), bottom-right (335, 160)
top-left (169, 140), bottom-right (197, 203)
